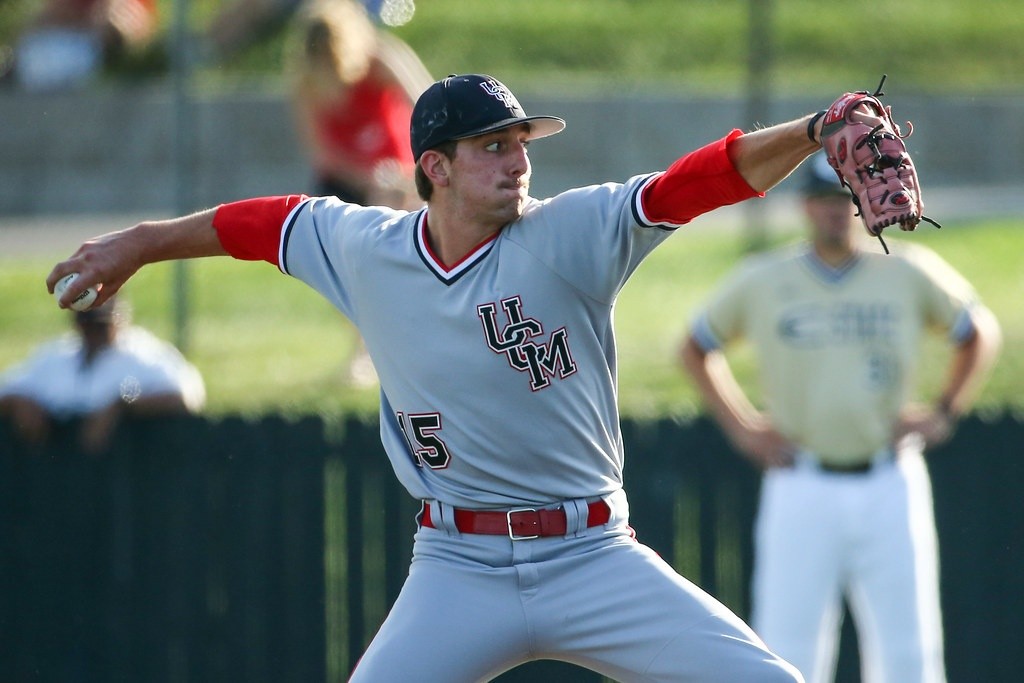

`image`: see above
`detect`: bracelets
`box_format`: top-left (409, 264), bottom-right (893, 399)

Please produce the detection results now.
top-left (807, 108), bottom-right (830, 145)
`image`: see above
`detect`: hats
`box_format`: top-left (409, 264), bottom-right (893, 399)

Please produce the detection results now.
top-left (410, 73), bottom-right (566, 164)
top-left (797, 151), bottom-right (852, 199)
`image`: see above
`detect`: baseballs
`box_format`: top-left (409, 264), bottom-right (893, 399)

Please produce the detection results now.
top-left (54, 272), bottom-right (100, 313)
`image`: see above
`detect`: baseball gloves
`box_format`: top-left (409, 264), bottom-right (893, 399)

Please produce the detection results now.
top-left (818, 89), bottom-right (926, 239)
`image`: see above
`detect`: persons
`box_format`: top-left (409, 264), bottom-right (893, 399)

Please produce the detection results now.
top-left (677, 147), bottom-right (1005, 683)
top-left (1, 276), bottom-right (210, 457)
top-left (280, 1), bottom-right (441, 394)
top-left (46, 72), bottom-right (892, 683)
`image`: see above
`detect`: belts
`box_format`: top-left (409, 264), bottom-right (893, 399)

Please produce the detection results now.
top-left (819, 459), bottom-right (873, 474)
top-left (419, 500), bottom-right (610, 542)
